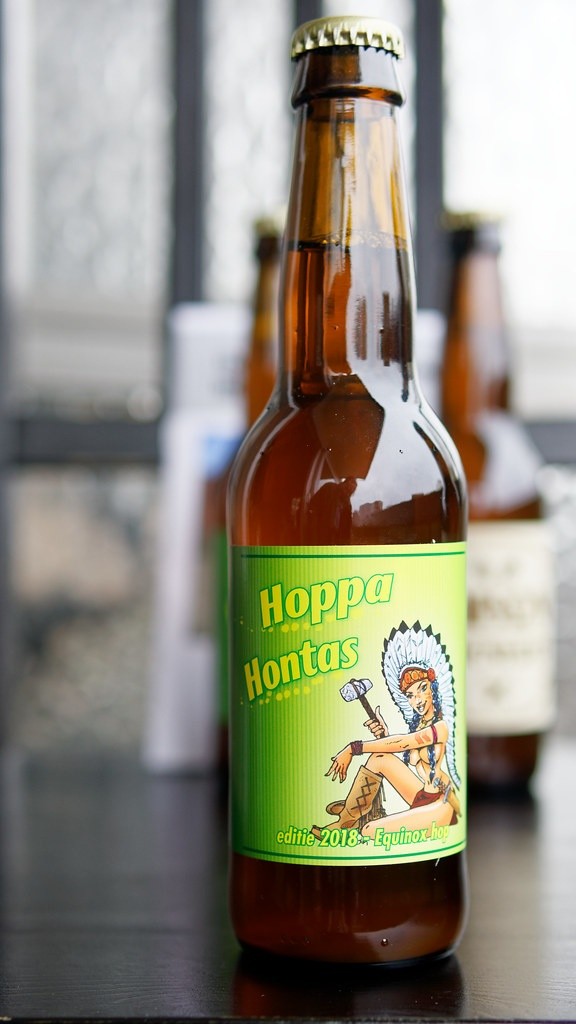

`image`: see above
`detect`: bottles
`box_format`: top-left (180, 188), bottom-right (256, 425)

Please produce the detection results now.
top-left (440, 209), bottom-right (558, 798)
top-left (223, 18), bottom-right (466, 981)
top-left (140, 301), bottom-right (251, 772)
top-left (204, 216), bottom-right (292, 817)
